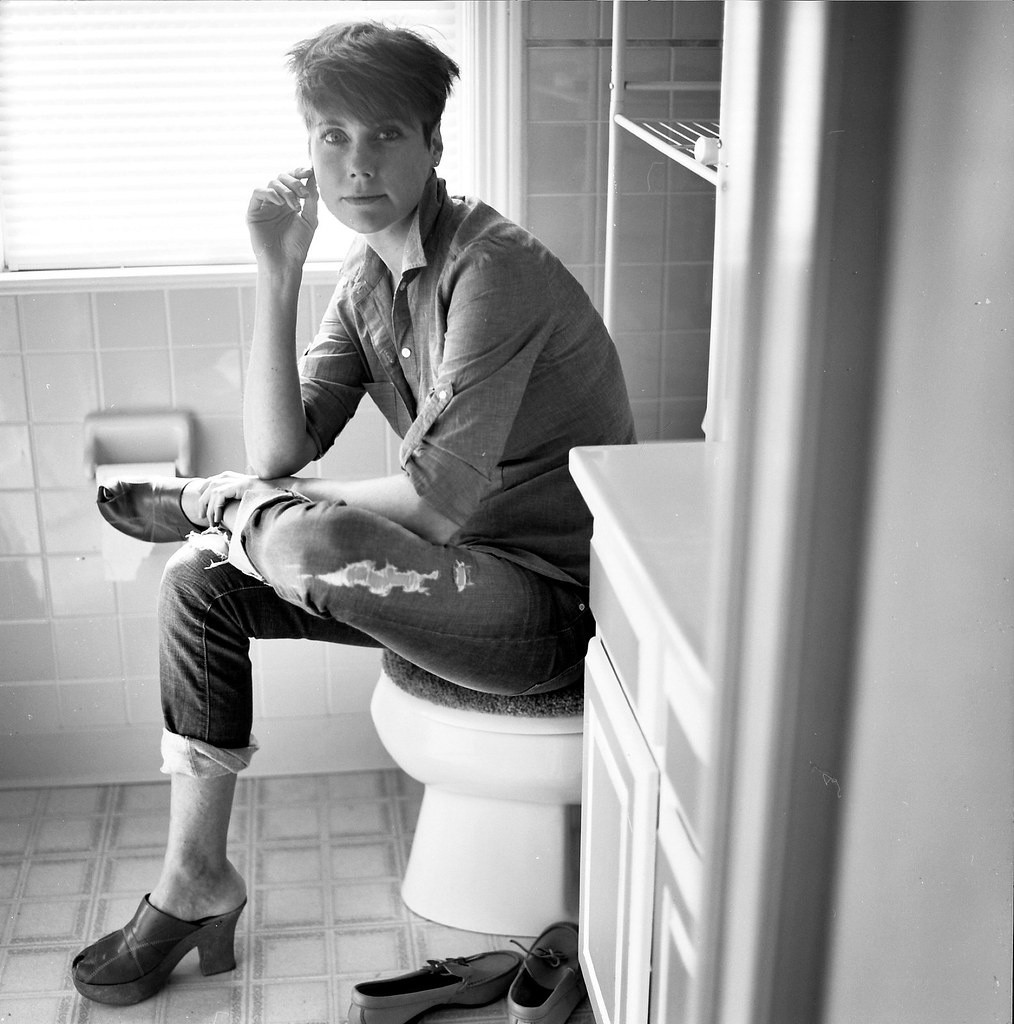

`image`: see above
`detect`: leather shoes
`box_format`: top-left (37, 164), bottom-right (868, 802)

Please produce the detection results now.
top-left (506, 922), bottom-right (587, 1024)
top-left (346, 950), bottom-right (524, 1024)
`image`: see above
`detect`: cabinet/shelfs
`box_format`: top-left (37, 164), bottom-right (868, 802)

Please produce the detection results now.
top-left (568, 441), bottom-right (721, 1024)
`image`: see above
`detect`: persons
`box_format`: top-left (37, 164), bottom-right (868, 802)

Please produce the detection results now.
top-left (72, 21), bottom-right (639, 1005)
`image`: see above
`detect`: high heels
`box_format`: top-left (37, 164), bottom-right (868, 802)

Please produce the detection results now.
top-left (96, 477), bottom-right (207, 543)
top-left (72, 893), bottom-right (248, 1006)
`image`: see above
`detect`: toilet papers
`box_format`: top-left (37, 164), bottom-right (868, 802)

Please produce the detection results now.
top-left (93, 462), bottom-right (176, 582)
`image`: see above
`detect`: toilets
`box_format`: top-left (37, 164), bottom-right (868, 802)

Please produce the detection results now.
top-left (370, 646), bottom-right (584, 938)
